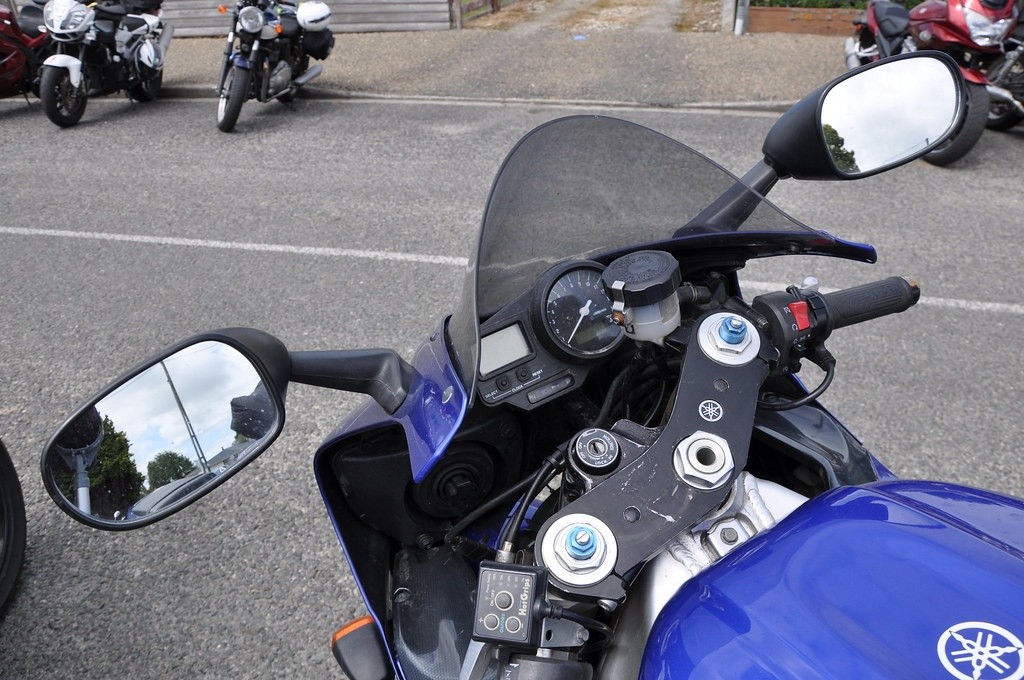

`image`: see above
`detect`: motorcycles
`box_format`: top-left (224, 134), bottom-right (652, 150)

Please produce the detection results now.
top-left (846, 0), bottom-right (1024, 167)
top-left (35, 48), bottom-right (1024, 679)
top-left (0, 0), bottom-right (176, 129)
top-left (214, 0), bottom-right (333, 132)
